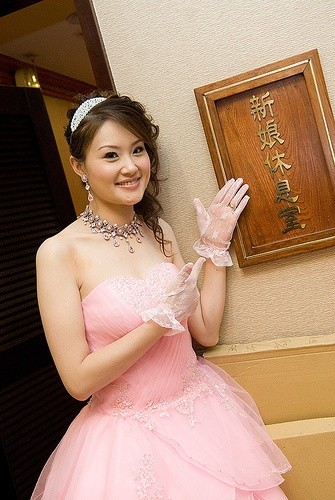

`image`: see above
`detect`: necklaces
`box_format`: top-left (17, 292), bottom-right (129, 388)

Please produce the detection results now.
top-left (78, 204), bottom-right (144, 253)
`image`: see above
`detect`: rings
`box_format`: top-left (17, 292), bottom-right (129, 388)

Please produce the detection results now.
top-left (229, 204), bottom-right (234, 210)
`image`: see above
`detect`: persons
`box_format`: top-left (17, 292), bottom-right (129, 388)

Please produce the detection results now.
top-left (32, 92), bottom-right (293, 500)
